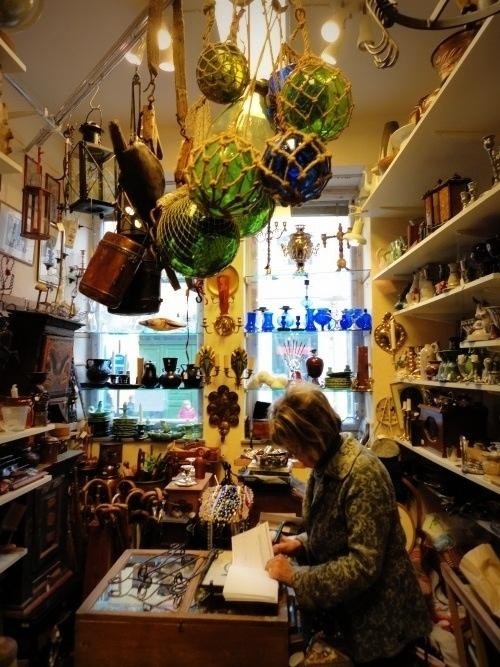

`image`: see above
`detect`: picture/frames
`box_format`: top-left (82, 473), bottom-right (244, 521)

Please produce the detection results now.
top-left (0, 155), bottom-right (65, 290)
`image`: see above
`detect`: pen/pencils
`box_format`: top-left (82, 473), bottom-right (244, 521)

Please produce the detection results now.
top-left (273, 520), bottom-right (285, 545)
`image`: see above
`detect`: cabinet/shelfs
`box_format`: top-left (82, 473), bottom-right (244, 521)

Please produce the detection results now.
top-left (358, 18), bottom-right (499, 666)
top-left (75, 434), bottom-right (318, 519)
top-left (1, 305), bottom-right (86, 667)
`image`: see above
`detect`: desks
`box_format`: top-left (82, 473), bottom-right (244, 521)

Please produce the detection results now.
top-left (77, 546), bottom-right (289, 667)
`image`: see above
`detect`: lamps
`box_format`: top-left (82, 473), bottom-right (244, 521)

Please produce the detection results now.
top-left (320, 6), bottom-right (372, 68)
top-left (122, 15), bottom-right (179, 74)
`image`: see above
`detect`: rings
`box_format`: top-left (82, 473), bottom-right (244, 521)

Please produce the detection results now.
top-left (268, 567), bottom-right (271, 571)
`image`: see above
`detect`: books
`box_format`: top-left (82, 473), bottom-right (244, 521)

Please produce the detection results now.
top-left (221, 519), bottom-right (280, 605)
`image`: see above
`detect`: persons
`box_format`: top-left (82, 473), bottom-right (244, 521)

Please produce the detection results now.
top-left (263, 386), bottom-right (434, 665)
top-left (177, 399), bottom-right (196, 419)
